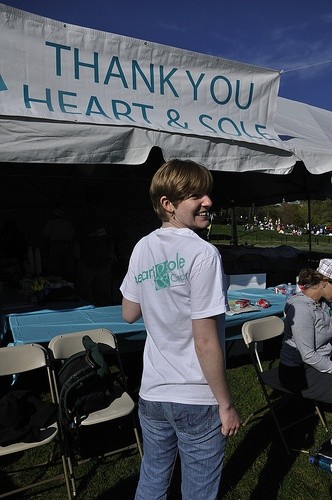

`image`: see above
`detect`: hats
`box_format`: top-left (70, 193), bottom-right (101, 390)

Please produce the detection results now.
top-left (316, 258), bottom-right (332, 278)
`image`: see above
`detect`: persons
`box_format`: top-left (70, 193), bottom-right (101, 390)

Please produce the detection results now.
top-left (243, 216), bottom-right (332, 237)
top-left (119, 158), bottom-right (241, 500)
top-left (279, 258), bottom-right (332, 459)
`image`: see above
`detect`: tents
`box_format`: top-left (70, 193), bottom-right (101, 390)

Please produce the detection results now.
top-left (0, 3), bottom-right (332, 255)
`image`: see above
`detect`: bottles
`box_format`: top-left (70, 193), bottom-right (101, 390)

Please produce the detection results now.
top-left (286, 283), bottom-right (293, 298)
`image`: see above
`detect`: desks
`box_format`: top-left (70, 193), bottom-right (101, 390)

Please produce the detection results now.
top-left (8, 285), bottom-right (296, 387)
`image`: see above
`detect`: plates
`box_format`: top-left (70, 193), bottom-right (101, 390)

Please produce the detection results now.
top-left (225, 300), bottom-right (260, 316)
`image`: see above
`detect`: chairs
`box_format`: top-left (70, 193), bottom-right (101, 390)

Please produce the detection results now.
top-left (0, 342), bottom-right (72, 500)
top-left (49, 327), bottom-right (142, 495)
top-left (240, 316), bottom-right (327, 461)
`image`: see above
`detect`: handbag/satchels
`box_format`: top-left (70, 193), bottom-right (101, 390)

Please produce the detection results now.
top-left (57, 335), bottom-right (125, 416)
